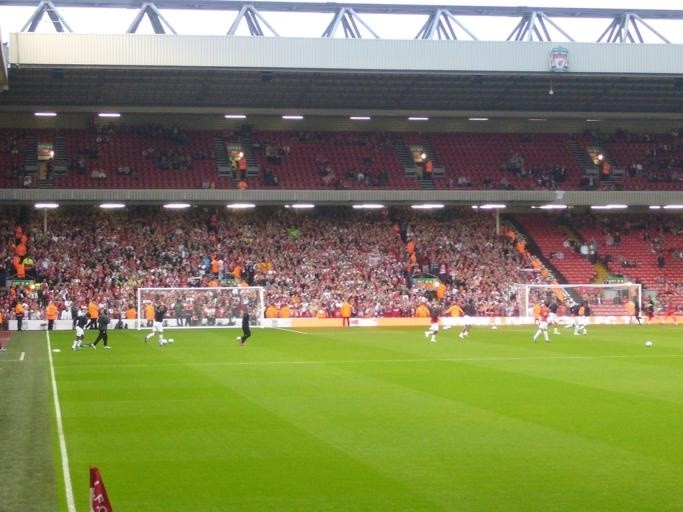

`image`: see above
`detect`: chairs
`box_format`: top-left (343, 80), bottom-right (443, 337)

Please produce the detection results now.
top-left (507, 210), bottom-right (682, 317)
top-left (3, 126), bottom-right (679, 191)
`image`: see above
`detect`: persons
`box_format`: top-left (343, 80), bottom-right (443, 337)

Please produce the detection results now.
top-left (1, 123), bottom-right (683, 191)
top-left (1, 203), bottom-right (683, 349)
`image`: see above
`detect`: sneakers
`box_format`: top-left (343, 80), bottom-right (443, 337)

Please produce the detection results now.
top-left (91, 344), bottom-right (111, 349)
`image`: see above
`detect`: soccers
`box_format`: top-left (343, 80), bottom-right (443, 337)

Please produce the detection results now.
top-left (645, 341), bottom-right (652, 348)
top-left (162, 338), bottom-right (174, 344)
